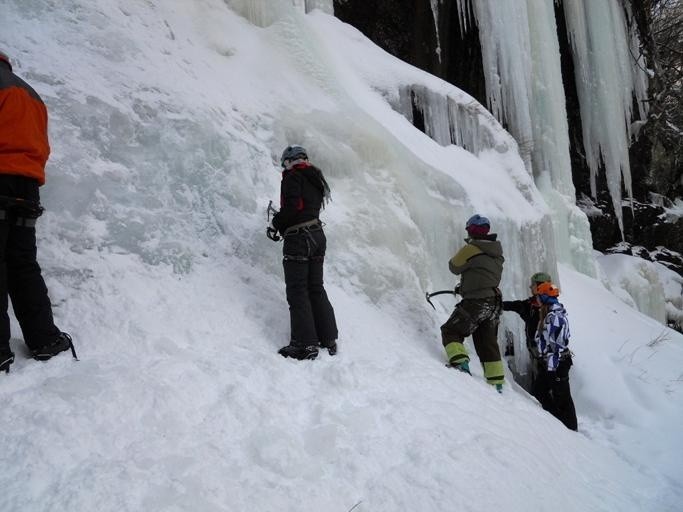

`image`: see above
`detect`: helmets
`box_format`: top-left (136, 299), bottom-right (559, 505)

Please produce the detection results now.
top-left (281, 146), bottom-right (308, 167)
top-left (465, 214), bottom-right (490, 228)
top-left (534, 283), bottom-right (559, 298)
top-left (531, 272), bottom-right (551, 283)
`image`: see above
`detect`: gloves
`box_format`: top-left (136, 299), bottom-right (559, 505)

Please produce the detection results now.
top-left (546, 371), bottom-right (557, 389)
top-left (273, 213), bottom-right (283, 230)
top-left (455, 283), bottom-right (462, 294)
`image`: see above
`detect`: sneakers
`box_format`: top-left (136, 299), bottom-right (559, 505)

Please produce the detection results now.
top-left (0, 333), bottom-right (71, 372)
top-left (279, 340), bottom-right (336, 359)
top-left (490, 384), bottom-right (503, 393)
top-left (451, 364), bottom-right (471, 375)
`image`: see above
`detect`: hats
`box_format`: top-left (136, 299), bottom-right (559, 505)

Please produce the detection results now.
top-left (0, 50), bottom-right (12, 70)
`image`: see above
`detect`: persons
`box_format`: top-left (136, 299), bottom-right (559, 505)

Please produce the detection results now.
top-left (530, 281), bottom-right (576, 434)
top-left (265, 144), bottom-right (340, 362)
top-left (500, 271), bottom-right (552, 382)
top-left (0, 52), bottom-right (73, 370)
top-left (438, 212), bottom-right (506, 396)
